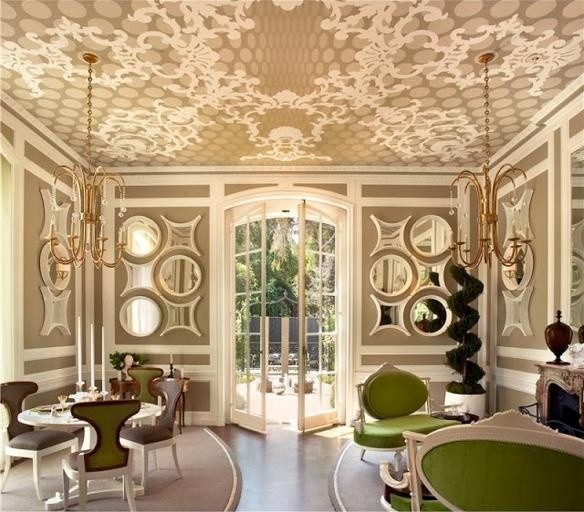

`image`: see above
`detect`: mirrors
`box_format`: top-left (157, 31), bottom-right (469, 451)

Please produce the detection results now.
top-left (117, 215), bottom-right (204, 341)
top-left (566, 147), bottom-right (584, 362)
top-left (366, 213), bottom-right (453, 340)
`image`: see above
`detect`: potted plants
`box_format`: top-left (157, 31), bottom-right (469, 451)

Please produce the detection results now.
top-left (442, 262), bottom-right (488, 417)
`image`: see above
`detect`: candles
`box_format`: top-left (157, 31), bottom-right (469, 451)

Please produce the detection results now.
top-left (169, 352), bottom-right (173, 364)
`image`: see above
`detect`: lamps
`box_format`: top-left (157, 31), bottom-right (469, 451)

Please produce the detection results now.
top-left (444, 54), bottom-right (533, 274)
top-left (543, 312), bottom-right (574, 365)
top-left (46, 52), bottom-right (128, 273)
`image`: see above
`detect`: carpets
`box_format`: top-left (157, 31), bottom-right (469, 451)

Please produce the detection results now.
top-left (1, 427), bottom-right (243, 512)
top-left (329, 431), bottom-right (434, 512)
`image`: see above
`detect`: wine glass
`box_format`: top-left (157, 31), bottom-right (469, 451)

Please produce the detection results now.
top-left (58, 395), bottom-right (67, 416)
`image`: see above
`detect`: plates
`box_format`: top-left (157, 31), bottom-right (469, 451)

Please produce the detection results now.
top-left (38, 404), bottom-right (70, 411)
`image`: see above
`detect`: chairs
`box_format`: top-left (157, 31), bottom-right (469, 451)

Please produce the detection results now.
top-left (0, 368), bottom-right (190, 511)
top-left (351, 363), bottom-right (583, 512)
